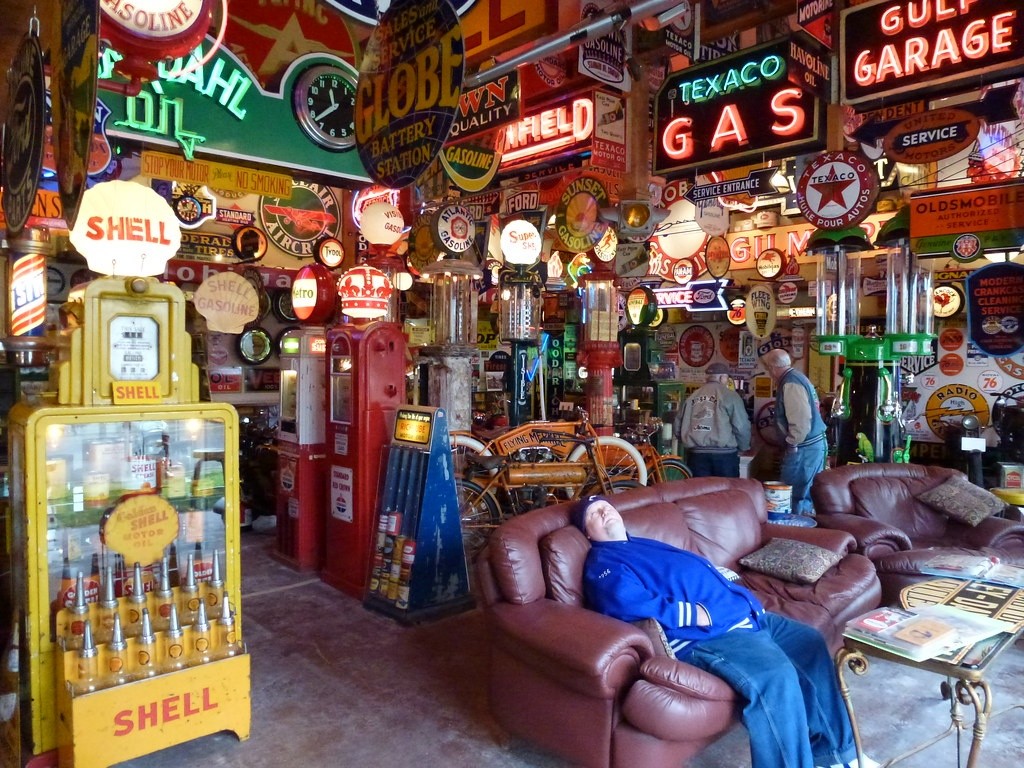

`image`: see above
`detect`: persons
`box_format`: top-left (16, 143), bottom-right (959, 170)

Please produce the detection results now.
top-left (569, 495), bottom-right (881, 768)
top-left (673, 364), bottom-right (751, 477)
top-left (762, 349), bottom-right (828, 516)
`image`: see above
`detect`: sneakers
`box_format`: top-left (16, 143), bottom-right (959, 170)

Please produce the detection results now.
top-left (816, 753), bottom-right (883, 768)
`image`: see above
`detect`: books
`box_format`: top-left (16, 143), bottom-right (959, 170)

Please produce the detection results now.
top-left (842, 600), bottom-right (1015, 663)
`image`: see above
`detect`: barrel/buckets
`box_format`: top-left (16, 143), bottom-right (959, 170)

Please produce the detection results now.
top-left (764, 481), bottom-right (792, 513)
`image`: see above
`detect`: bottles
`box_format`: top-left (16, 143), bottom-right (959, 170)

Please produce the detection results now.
top-left (155, 557), bottom-right (174, 629)
top-left (218, 592), bottom-right (237, 657)
top-left (193, 598), bottom-right (212, 664)
top-left (165, 603), bottom-right (184, 670)
top-left (114, 553), bottom-right (128, 598)
top-left (128, 562), bottom-right (147, 635)
top-left (78, 620), bottom-right (98, 693)
top-left (99, 567), bottom-right (118, 642)
top-left (180, 555), bottom-right (198, 623)
top-left (156, 435), bottom-right (171, 488)
top-left (169, 545), bottom-right (180, 587)
top-left (58, 558), bottom-right (71, 595)
top-left (193, 542), bottom-right (203, 564)
top-left (141, 608), bottom-right (157, 678)
top-left (87, 553), bottom-right (100, 603)
top-left (69, 572), bottom-right (89, 648)
top-left (106, 613), bottom-right (127, 686)
top-left (208, 550), bottom-right (224, 618)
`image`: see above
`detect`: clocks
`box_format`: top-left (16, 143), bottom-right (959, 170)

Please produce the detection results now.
top-left (290, 63), bottom-right (358, 154)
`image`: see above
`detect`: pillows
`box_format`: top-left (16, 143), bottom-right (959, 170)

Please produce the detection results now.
top-left (914, 473), bottom-right (1010, 527)
top-left (738, 537), bottom-right (843, 584)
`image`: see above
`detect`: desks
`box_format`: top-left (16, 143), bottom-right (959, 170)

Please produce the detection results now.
top-left (834, 603), bottom-right (1023, 768)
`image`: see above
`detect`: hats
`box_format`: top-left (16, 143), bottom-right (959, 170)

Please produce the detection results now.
top-left (570, 494), bottom-right (615, 532)
top-left (705, 363), bottom-right (730, 374)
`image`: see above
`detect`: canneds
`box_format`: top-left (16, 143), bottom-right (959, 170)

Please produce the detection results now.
top-left (370, 511), bottom-right (416, 609)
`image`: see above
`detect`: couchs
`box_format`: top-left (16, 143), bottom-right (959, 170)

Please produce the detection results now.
top-left (474, 478), bottom-right (881, 767)
top-left (810, 462), bottom-right (1024, 602)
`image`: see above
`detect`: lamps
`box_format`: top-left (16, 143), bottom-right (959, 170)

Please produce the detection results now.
top-left (673, 258), bottom-right (699, 286)
top-left (499, 219), bottom-right (541, 282)
top-left (649, 308), bottom-right (668, 329)
top-left (933, 284), bottom-right (964, 320)
top-left (756, 247), bottom-right (787, 280)
top-left (313, 236), bottom-right (346, 272)
top-left (291, 262), bottom-right (335, 326)
top-left (272, 288), bottom-right (300, 323)
top-left (275, 326), bottom-right (299, 356)
top-left (335, 264), bottom-right (393, 318)
top-left (173, 195), bottom-right (202, 225)
top-left (231, 225), bottom-right (268, 262)
top-left (625, 286), bottom-right (658, 327)
top-left (725, 296), bottom-right (746, 327)
top-left (98, 0), bottom-right (227, 97)
top-left (429, 203), bottom-right (476, 259)
top-left (1, 224), bottom-right (56, 365)
top-left (360, 201), bottom-right (404, 266)
top-left (69, 179), bottom-right (182, 277)
top-left (235, 326), bottom-right (274, 365)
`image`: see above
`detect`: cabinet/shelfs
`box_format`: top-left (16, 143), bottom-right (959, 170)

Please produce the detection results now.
top-left (9, 398), bottom-right (240, 761)
top-left (56, 584), bottom-right (251, 768)
